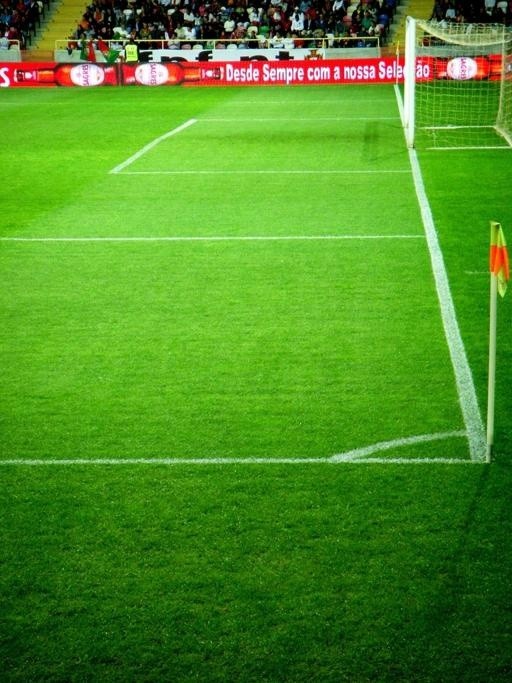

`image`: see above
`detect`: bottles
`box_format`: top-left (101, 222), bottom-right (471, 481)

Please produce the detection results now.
top-left (13, 63), bottom-right (223, 86)
top-left (358, 39), bottom-right (365, 48)
top-left (125, 38), bottom-right (139, 61)
top-left (433, 55), bottom-right (512, 80)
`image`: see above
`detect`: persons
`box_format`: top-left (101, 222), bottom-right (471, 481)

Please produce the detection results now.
top-left (1, 1), bottom-right (511, 50)
top-left (124, 37), bottom-right (140, 64)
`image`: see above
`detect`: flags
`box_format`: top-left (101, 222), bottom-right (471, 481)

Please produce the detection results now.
top-left (81, 41), bottom-right (97, 64)
top-left (97, 37), bottom-right (119, 64)
